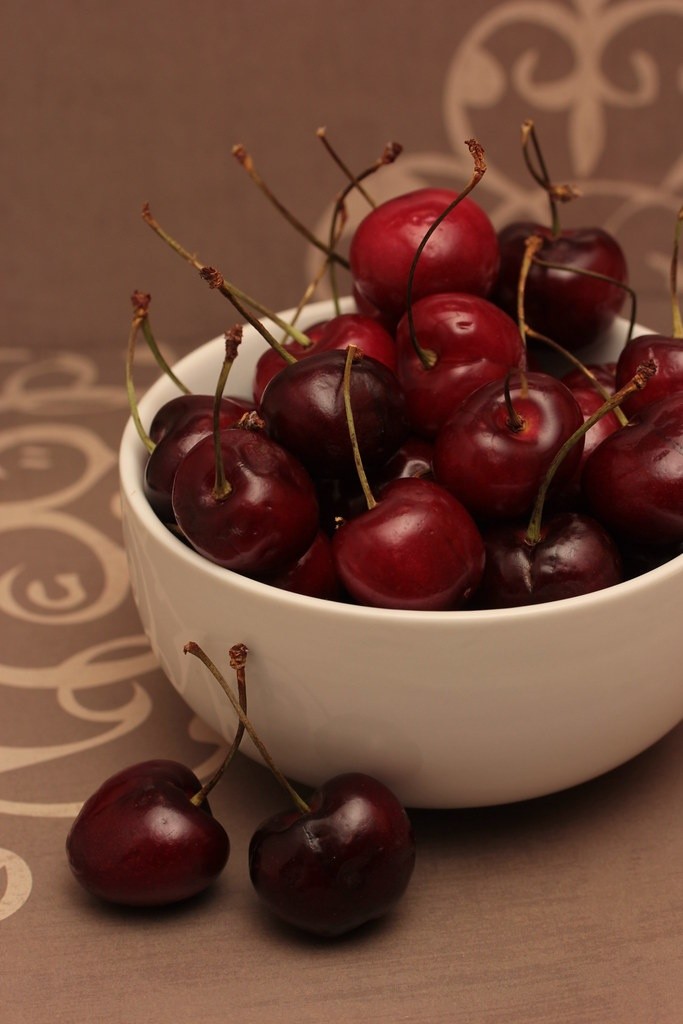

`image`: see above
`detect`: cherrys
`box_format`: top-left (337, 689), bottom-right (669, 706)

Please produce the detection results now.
top-left (121, 117), bottom-right (683, 612)
top-left (65, 644), bottom-right (248, 913)
top-left (180, 640), bottom-right (414, 936)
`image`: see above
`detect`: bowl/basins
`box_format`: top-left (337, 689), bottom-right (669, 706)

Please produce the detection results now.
top-left (120, 295), bottom-right (683, 805)
